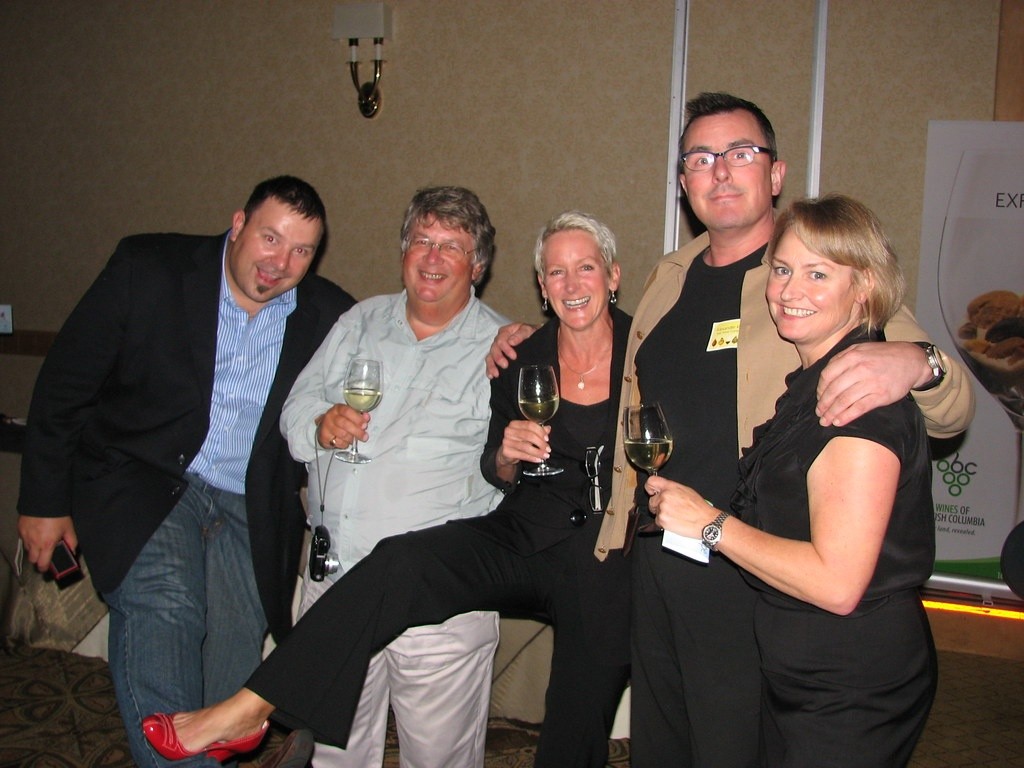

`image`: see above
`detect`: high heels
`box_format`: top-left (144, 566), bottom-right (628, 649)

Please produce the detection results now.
top-left (142, 712), bottom-right (269, 761)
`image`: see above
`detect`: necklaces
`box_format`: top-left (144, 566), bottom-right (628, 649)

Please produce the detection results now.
top-left (558, 327), bottom-right (609, 389)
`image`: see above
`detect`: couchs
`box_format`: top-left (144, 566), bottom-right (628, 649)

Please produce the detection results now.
top-left (6, 536), bottom-right (632, 745)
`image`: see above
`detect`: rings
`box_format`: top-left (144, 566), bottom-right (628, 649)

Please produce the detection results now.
top-left (329, 436), bottom-right (336, 446)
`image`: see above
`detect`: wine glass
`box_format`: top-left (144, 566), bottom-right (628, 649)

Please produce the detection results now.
top-left (622, 402), bottom-right (674, 533)
top-left (938, 144), bottom-right (1023, 578)
top-left (518, 364), bottom-right (565, 476)
top-left (334, 358), bottom-right (384, 465)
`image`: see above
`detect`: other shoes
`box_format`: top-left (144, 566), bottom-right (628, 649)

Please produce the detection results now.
top-left (242, 728), bottom-right (314, 768)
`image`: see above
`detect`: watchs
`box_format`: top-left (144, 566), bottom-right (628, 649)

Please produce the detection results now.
top-left (702, 511), bottom-right (730, 552)
top-left (912, 339), bottom-right (947, 392)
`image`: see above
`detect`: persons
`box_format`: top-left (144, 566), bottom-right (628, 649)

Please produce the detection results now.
top-left (643, 190), bottom-right (937, 768)
top-left (17, 174), bottom-right (361, 768)
top-left (486, 91), bottom-right (976, 768)
top-left (142, 186), bottom-right (635, 768)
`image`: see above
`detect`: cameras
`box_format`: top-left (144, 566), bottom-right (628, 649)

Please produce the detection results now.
top-left (48, 539), bottom-right (78, 580)
top-left (309, 525), bottom-right (339, 582)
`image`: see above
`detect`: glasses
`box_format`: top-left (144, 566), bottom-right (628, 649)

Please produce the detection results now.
top-left (409, 238), bottom-right (478, 261)
top-left (680, 146), bottom-right (775, 171)
